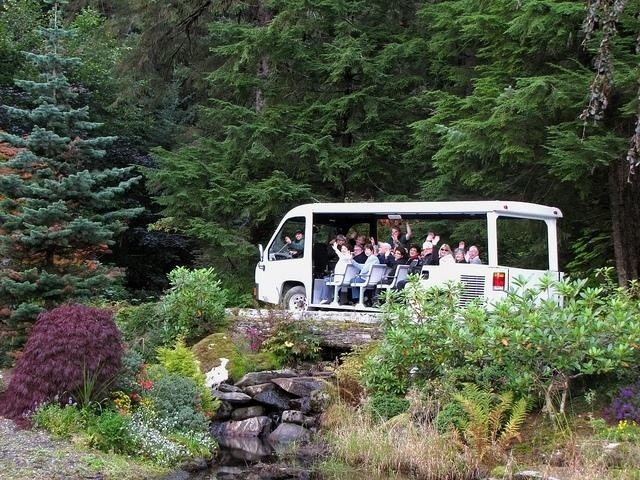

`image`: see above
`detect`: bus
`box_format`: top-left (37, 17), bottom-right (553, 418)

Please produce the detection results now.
top-left (253, 200), bottom-right (564, 318)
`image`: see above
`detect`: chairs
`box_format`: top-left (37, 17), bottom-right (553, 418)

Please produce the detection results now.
top-left (377, 264), bottom-right (412, 302)
top-left (325, 264), bottom-right (361, 306)
top-left (350, 263), bottom-right (388, 307)
top-left (383, 267), bottom-right (393, 279)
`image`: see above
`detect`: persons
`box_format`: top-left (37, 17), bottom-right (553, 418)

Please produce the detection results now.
top-left (284, 219), bottom-right (484, 308)
